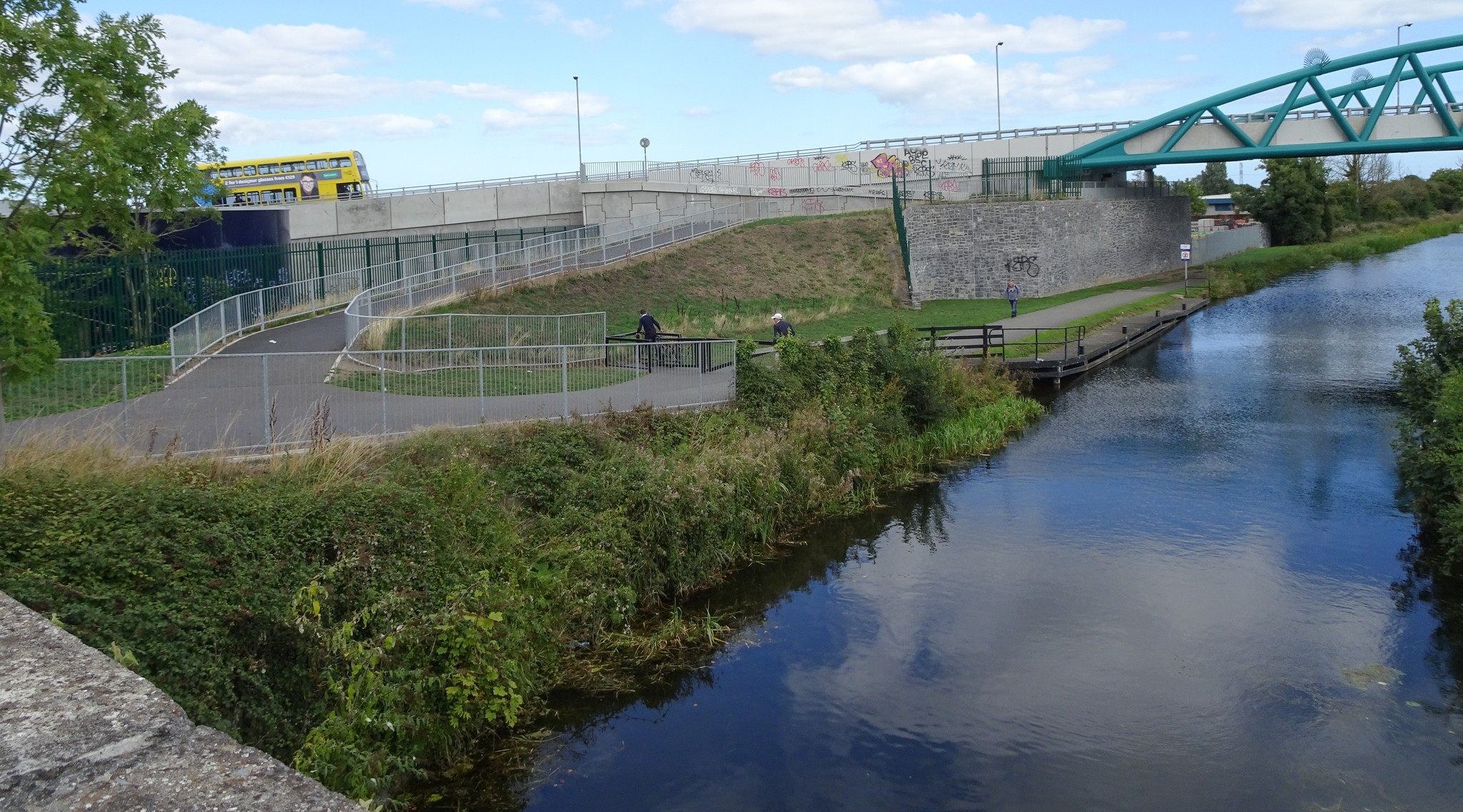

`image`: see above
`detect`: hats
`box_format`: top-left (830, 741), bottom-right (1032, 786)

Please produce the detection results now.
top-left (771, 313), bottom-right (783, 320)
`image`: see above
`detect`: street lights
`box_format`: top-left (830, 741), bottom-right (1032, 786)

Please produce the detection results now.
top-left (573, 74), bottom-right (582, 165)
top-left (995, 40), bottom-right (1005, 131)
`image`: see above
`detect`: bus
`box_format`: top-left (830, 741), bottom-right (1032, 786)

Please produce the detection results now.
top-left (197, 150), bottom-right (374, 207)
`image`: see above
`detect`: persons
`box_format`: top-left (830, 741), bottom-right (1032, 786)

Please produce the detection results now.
top-left (1006, 281), bottom-right (1021, 318)
top-left (233, 196), bottom-right (253, 207)
top-left (299, 172), bottom-right (319, 200)
top-left (336, 159), bottom-right (341, 168)
top-left (343, 185), bottom-right (351, 197)
top-left (637, 309), bottom-right (663, 367)
top-left (771, 313), bottom-right (795, 346)
